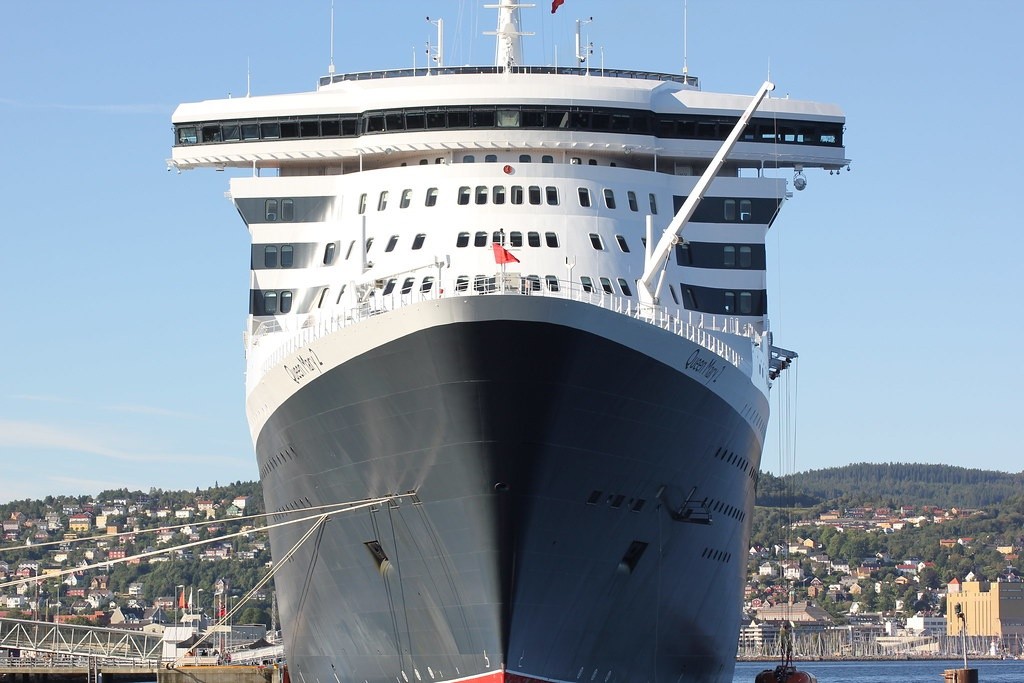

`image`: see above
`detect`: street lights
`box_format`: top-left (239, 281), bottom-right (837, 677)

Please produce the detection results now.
top-left (229, 595), bottom-right (239, 654)
top-left (174, 585), bottom-right (185, 661)
top-left (54, 584), bottom-right (59, 651)
top-left (196, 589), bottom-right (204, 638)
top-left (213, 592), bottom-right (220, 653)
top-left (158, 596), bottom-right (163, 624)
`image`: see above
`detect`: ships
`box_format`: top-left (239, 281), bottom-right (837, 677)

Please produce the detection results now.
top-left (169, 1), bottom-right (853, 683)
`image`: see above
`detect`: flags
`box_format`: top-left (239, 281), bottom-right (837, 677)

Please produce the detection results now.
top-left (492, 243), bottom-right (520, 264)
top-left (551, 0), bottom-right (564, 13)
